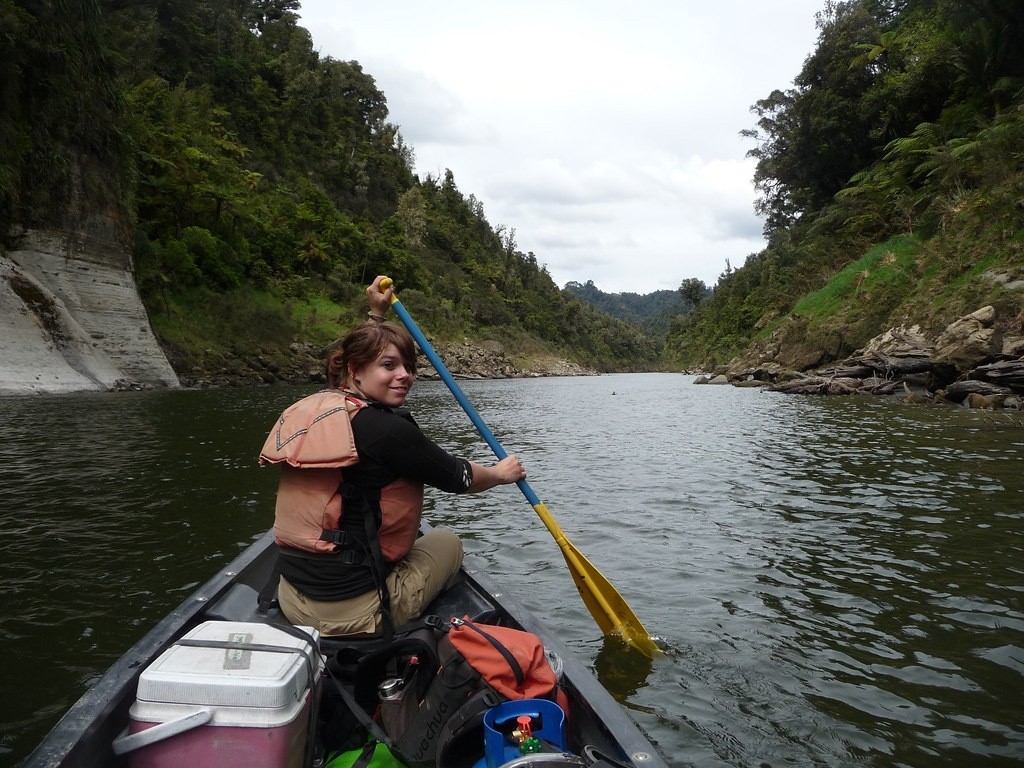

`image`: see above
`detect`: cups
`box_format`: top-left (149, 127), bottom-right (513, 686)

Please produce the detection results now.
top-left (377, 679), bottom-right (404, 701)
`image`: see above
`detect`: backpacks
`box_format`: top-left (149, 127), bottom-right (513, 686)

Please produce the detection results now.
top-left (326, 615), bottom-right (569, 768)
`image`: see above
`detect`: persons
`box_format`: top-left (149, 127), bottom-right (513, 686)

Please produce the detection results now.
top-left (258, 276), bottom-right (528, 637)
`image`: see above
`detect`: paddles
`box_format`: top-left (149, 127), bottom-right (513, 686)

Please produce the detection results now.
top-left (380, 278), bottom-right (664, 659)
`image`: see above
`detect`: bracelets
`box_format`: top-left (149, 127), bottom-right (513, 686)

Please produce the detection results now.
top-left (368, 310), bottom-right (387, 323)
